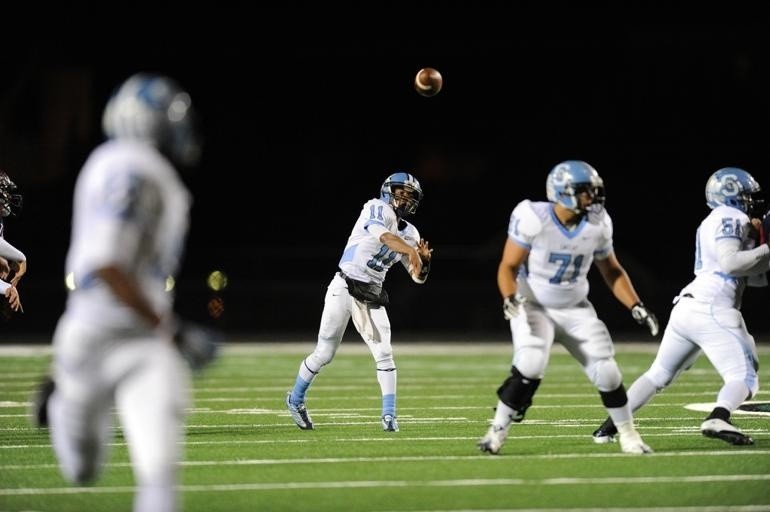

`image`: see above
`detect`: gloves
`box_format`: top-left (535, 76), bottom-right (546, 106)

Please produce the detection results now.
top-left (503, 294), bottom-right (523, 323)
top-left (630, 301), bottom-right (660, 337)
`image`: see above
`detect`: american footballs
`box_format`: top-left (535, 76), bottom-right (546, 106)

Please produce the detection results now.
top-left (415, 68), bottom-right (442, 98)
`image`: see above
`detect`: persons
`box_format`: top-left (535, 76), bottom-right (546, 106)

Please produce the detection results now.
top-left (285, 172), bottom-right (432, 432)
top-left (593, 166), bottom-right (769, 445)
top-left (27, 71), bottom-right (202, 510)
top-left (477, 159), bottom-right (658, 453)
top-left (0, 172), bottom-right (27, 325)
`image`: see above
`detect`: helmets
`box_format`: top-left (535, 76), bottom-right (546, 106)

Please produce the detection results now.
top-left (0, 170), bottom-right (19, 221)
top-left (101, 68), bottom-right (197, 149)
top-left (379, 171), bottom-right (425, 215)
top-left (544, 159), bottom-right (607, 215)
top-left (704, 165), bottom-right (759, 211)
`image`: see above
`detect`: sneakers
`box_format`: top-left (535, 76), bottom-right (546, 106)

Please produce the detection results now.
top-left (284, 392), bottom-right (314, 431)
top-left (379, 413), bottom-right (399, 433)
top-left (476, 422), bottom-right (507, 455)
top-left (591, 427), bottom-right (618, 444)
top-left (699, 415), bottom-right (755, 447)
top-left (621, 434), bottom-right (653, 454)
